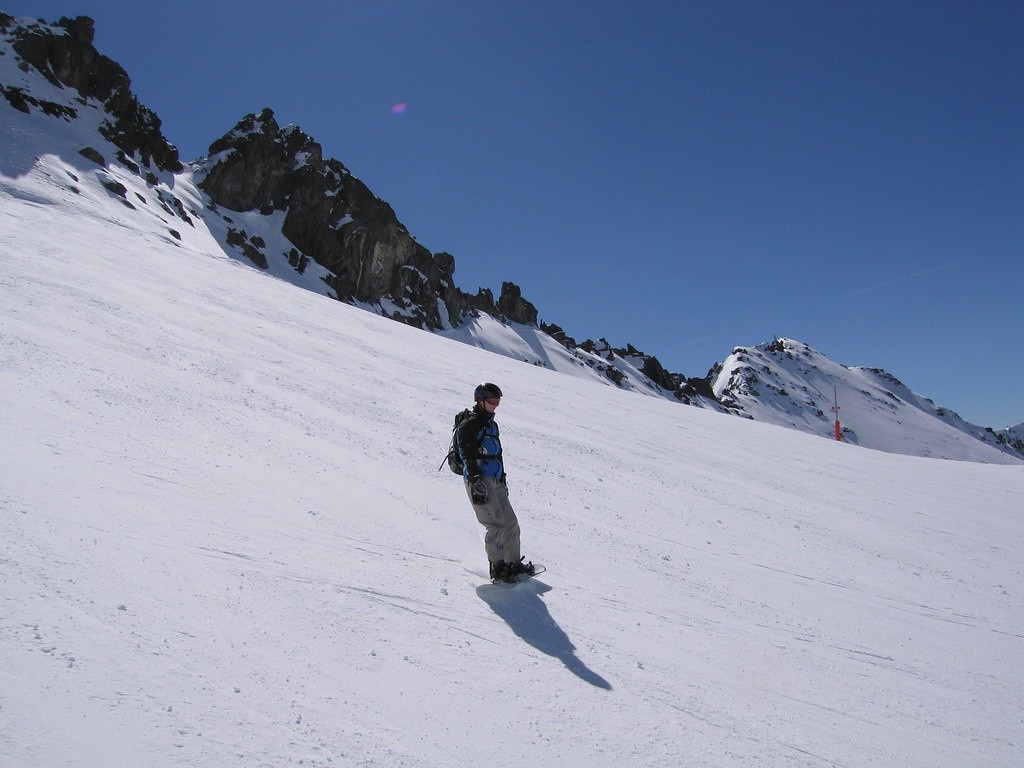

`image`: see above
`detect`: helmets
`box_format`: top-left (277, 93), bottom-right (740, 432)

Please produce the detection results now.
top-left (473, 383), bottom-right (503, 402)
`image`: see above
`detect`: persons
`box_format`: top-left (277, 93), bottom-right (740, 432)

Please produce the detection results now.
top-left (456, 383), bottom-right (535, 584)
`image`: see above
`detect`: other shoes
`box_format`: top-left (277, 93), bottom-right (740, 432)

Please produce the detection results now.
top-left (507, 561), bottom-right (535, 577)
top-left (489, 560), bottom-right (520, 584)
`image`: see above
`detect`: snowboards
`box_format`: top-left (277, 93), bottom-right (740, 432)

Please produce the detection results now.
top-left (492, 563), bottom-right (546, 589)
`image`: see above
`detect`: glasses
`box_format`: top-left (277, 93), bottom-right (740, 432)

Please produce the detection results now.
top-left (487, 399), bottom-right (500, 406)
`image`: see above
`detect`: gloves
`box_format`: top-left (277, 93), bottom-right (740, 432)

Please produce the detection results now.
top-left (466, 472), bottom-right (488, 505)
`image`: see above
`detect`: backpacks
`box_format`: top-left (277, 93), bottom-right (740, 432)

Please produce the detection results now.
top-left (439, 409), bottom-right (485, 475)
